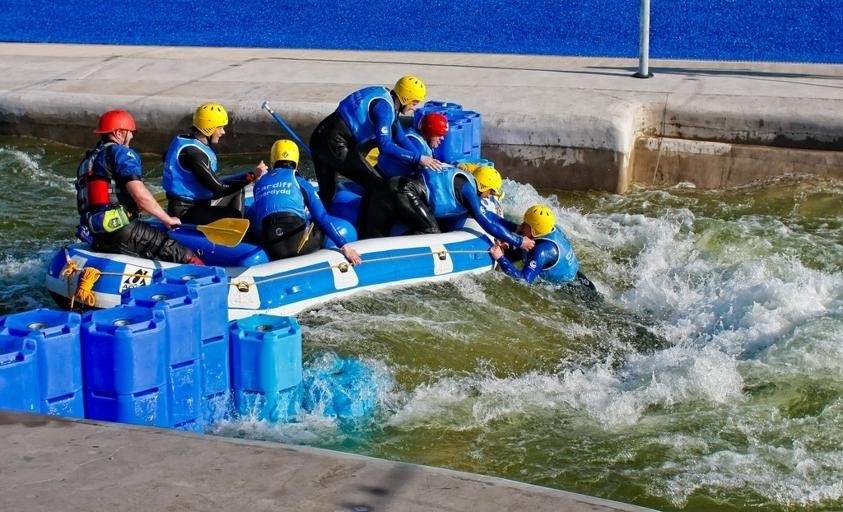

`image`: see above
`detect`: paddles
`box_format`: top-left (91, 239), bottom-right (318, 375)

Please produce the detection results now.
top-left (298, 220), bottom-right (314, 253)
top-left (138, 218), bottom-right (250, 247)
top-left (152, 167), bottom-right (257, 207)
top-left (366, 147), bottom-right (505, 202)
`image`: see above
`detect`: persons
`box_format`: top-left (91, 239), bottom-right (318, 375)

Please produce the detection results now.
top-left (377, 111), bottom-right (476, 177)
top-left (309, 74), bottom-right (442, 240)
top-left (75, 108), bottom-right (207, 266)
top-left (489, 203), bottom-right (598, 300)
top-left (252, 138), bottom-right (363, 267)
top-left (160, 101), bottom-right (269, 226)
top-left (373, 166), bottom-right (535, 255)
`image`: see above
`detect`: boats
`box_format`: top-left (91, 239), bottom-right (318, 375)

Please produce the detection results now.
top-left (45, 182), bottom-right (505, 328)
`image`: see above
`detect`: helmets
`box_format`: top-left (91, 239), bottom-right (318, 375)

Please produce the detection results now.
top-left (94, 110), bottom-right (136, 133)
top-left (418, 113), bottom-right (448, 139)
top-left (393, 76), bottom-right (426, 105)
top-left (270, 139), bottom-right (299, 170)
top-left (473, 166), bottom-right (502, 193)
top-left (524, 205), bottom-right (556, 237)
top-left (193, 103), bottom-right (228, 136)
